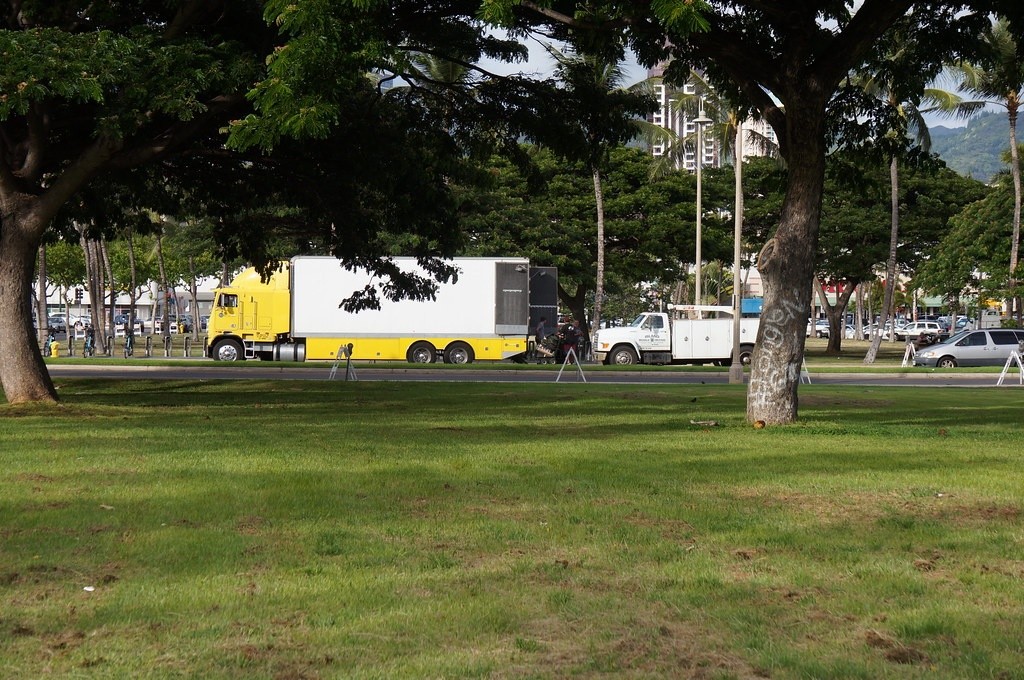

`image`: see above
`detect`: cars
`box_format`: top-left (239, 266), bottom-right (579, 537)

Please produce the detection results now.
top-left (32, 307), bottom-right (208, 336)
top-left (806, 312), bottom-right (974, 344)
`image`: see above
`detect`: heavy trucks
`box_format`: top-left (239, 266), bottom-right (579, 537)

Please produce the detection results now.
top-left (202, 256), bottom-right (559, 367)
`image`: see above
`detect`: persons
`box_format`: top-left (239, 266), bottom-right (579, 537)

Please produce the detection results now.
top-left (534, 317), bottom-right (547, 360)
top-left (560, 315), bottom-right (582, 365)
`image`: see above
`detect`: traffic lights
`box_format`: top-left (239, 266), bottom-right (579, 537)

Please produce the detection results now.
top-left (76, 289), bottom-right (84, 299)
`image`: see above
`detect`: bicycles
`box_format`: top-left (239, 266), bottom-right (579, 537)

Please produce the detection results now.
top-left (122, 328), bottom-right (139, 359)
top-left (43, 329), bottom-right (59, 356)
top-left (81, 324), bottom-right (95, 358)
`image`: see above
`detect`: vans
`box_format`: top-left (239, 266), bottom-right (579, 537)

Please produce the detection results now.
top-left (912, 328), bottom-right (1024, 367)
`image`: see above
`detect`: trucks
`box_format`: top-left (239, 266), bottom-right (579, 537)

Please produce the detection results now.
top-left (589, 304), bottom-right (761, 368)
